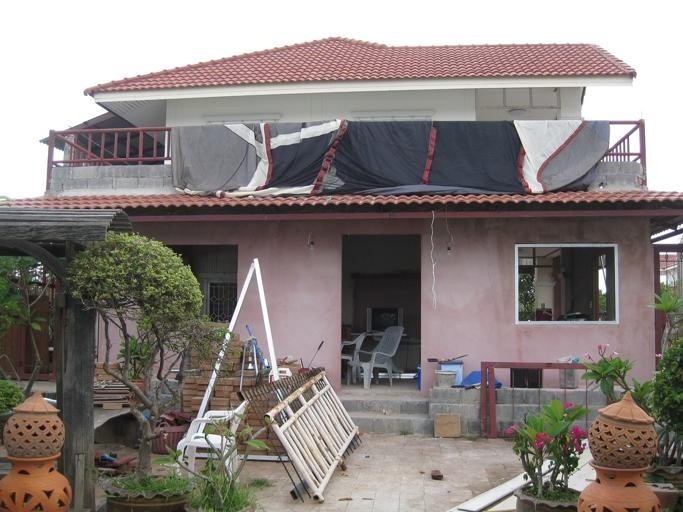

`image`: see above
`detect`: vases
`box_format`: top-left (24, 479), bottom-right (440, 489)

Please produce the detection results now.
top-left (514, 486), bottom-right (581, 512)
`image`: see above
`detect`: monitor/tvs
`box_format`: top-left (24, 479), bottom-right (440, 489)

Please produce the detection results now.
top-left (366, 303), bottom-right (404, 334)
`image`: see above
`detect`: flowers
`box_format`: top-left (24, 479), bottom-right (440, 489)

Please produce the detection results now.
top-left (507, 398), bottom-right (591, 499)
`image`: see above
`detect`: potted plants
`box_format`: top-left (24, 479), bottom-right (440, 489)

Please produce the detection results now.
top-left (63, 230), bottom-right (244, 512)
top-left (579, 290), bottom-right (683, 490)
top-left (150, 399), bottom-right (278, 512)
top-left (0, 256), bottom-right (53, 446)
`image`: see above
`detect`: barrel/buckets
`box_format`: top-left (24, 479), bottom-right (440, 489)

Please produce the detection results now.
top-left (558, 369), bottom-right (579, 389)
top-left (439, 360), bottom-right (464, 388)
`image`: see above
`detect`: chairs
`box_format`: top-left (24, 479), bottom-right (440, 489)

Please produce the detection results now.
top-left (176, 399), bottom-right (251, 483)
top-left (341, 326), bottom-right (404, 389)
